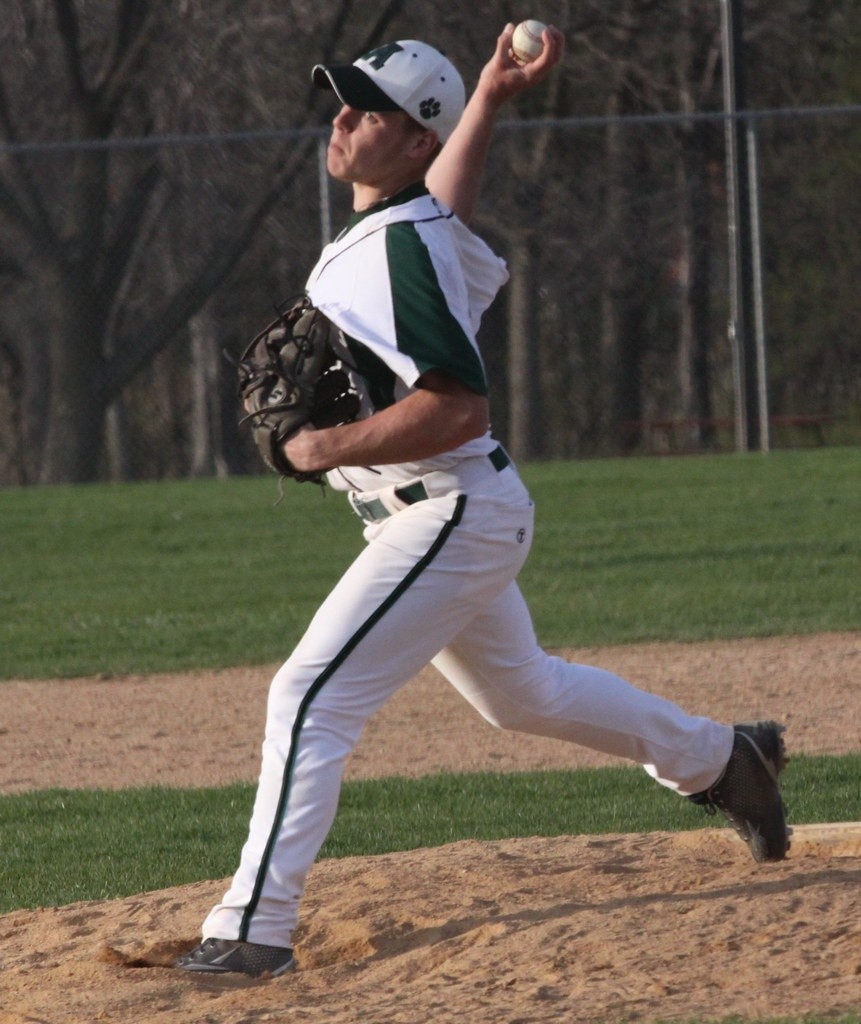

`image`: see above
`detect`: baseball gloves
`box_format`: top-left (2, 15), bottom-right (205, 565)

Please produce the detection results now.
top-left (233, 294), bottom-right (363, 483)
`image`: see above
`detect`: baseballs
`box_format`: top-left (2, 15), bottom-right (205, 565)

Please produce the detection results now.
top-left (511, 18), bottom-right (551, 64)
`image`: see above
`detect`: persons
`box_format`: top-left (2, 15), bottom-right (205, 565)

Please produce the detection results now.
top-left (171, 22), bottom-right (793, 979)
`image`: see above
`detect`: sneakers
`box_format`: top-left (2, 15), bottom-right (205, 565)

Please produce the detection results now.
top-left (173, 937), bottom-right (296, 977)
top-left (688, 721), bottom-right (793, 863)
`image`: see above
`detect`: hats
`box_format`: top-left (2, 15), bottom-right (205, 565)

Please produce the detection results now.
top-left (310, 39), bottom-right (466, 147)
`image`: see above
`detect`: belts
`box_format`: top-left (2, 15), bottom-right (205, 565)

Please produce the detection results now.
top-left (354, 445), bottom-right (510, 524)
